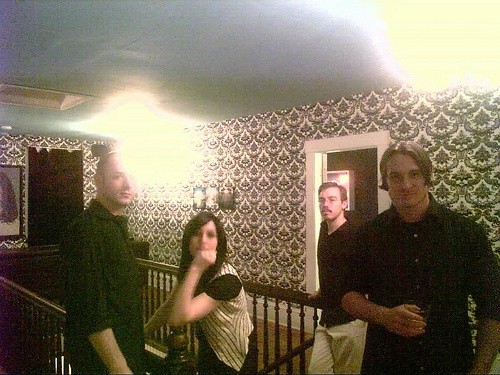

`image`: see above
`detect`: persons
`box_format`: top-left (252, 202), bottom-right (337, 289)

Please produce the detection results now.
top-left (336, 141), bottom-right (500, 375)
top-left (145, 211), bottom-right (258, 375)
top-left (308, 182), bottom-right (368, 375)
top-left (62, 152), bottom-right (146, 375)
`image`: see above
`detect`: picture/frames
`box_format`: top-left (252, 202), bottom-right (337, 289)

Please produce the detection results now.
top-left (0, 163), bottom-right (23, 242)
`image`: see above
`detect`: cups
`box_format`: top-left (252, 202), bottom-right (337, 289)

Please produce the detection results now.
top-left (404, 297), bottom-right (431, 321)
